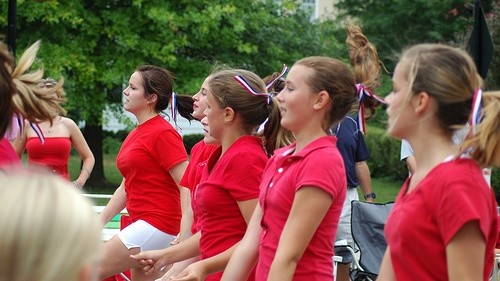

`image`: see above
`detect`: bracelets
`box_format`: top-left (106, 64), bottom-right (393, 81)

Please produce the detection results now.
top-left (364, 192), bottom-right (376, 199)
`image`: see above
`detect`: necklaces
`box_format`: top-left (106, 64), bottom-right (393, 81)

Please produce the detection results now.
top-left (40, 113), bottom-right (64, 137)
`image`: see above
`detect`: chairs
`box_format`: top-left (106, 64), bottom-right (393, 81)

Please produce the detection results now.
top-left (333, 200), bottom-right (395, 281)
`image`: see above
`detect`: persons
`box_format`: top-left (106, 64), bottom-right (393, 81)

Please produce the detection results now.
top-left (130, 62), bottom-right (281, 281)
top-left (99, 66), bottom-right (189, 281)
top-left (0, 39), bottom-right (101, 281)
top-left (220, 56), bottom-right (377, 281)
top-left (375, 44), bottom-right (499, 281)
